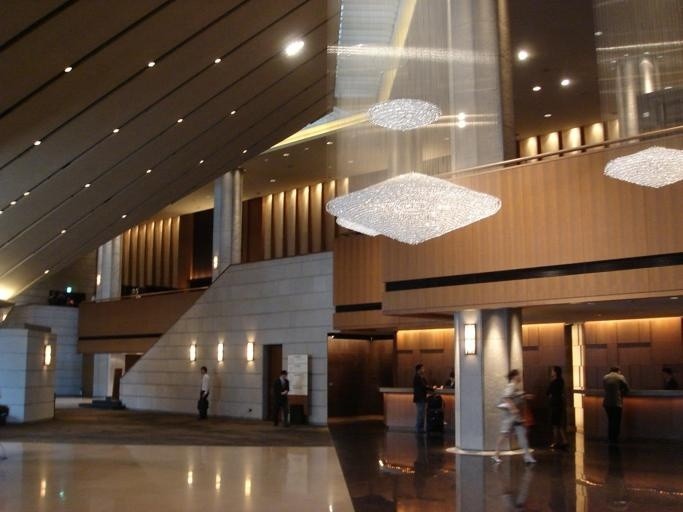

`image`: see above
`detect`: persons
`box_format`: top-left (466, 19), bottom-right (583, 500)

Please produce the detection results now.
top-left (274, 370), bottom-right (292, 427)
top-left (445, 371), bottom-right (455, 389)
top-left (546, 366), bottom-right (571, 448)
top-left (412, 362), bottom-right (444, 432)
top-left (489, 368), bottom-right (538, 464)
top-left (601, 365), bottom-right (631, 443)
top-left (661, 366), bottom-right (680, 390)
top-left (198, 366), bottom-right (211, 420)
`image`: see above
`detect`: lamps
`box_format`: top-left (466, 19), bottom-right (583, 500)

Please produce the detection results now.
top-left (322, 0), bottom-right (506, 245)
top-left (245, 342), bottom-right (253, 361)
top-left (591, 0), bottom-right (682, 189)
top-left (43, 344), bottom-right (51, 365)
top-left (187, 344), bottom-right (196, 362)
top-left (216, 343), bottom-right (223, 362)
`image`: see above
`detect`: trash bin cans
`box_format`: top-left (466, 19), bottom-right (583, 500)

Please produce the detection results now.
top-left (290, 404), bottom-right (304, 426)
